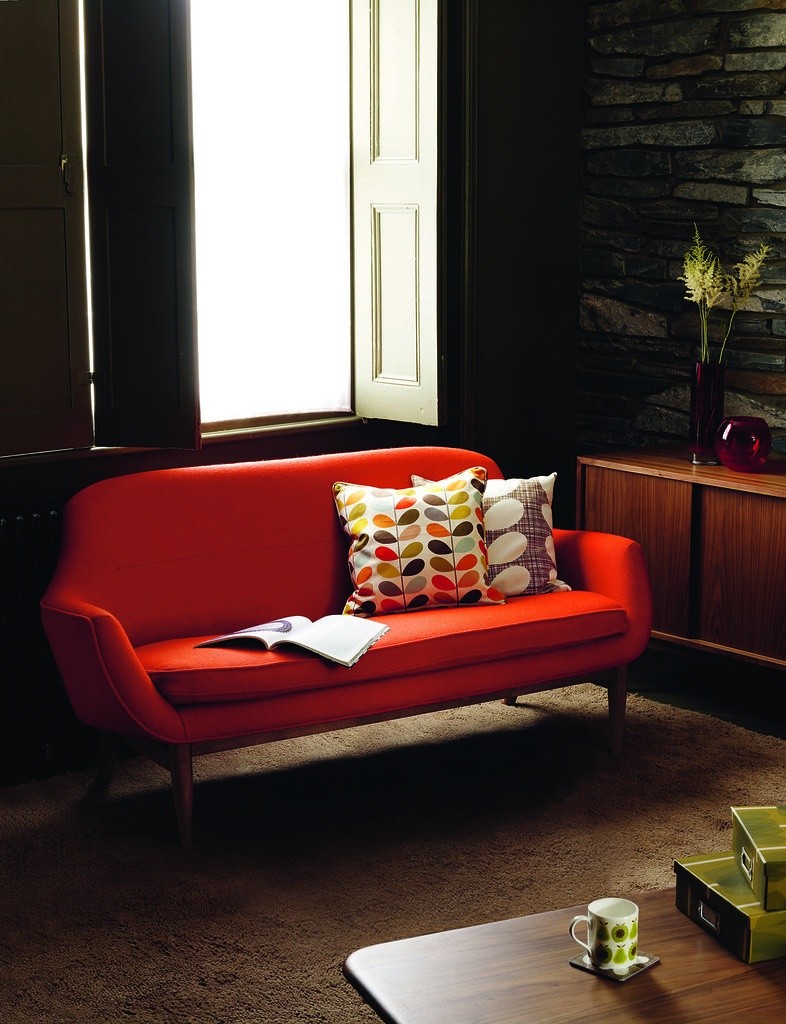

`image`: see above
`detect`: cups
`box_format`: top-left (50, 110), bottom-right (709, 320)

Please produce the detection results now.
top-left (568, 898), bottom-right (639, 968)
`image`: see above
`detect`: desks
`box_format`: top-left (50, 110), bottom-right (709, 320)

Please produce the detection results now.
top-left (345, 887), bottom-right (786, 1023)
top-left (570, 453), bottom-right (786, 674)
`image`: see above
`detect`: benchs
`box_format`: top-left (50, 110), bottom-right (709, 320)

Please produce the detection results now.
top-left (39, 445), bottom-right (654, 847)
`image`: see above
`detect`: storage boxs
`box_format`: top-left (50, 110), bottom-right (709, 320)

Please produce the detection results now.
top-left (730, 803), bottom-right (786, 911)
top-left (672, 853), bottom-right (786, 966)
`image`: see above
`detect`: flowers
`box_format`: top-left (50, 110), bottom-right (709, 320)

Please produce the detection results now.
top-left (672, 227), bottom-right (770, 362)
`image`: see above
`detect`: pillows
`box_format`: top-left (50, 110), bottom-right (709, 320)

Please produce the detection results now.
top-left (411, 473), bottom-right (572, 592)
top-left (331, 468), bottom-right (507, 611)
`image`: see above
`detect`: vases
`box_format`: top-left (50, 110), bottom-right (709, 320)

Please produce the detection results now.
top-left (682, 357), bottom-right (721, 467)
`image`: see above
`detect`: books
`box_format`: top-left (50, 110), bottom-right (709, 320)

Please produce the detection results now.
top-left (195, 615), bottom-right (390, 668)
